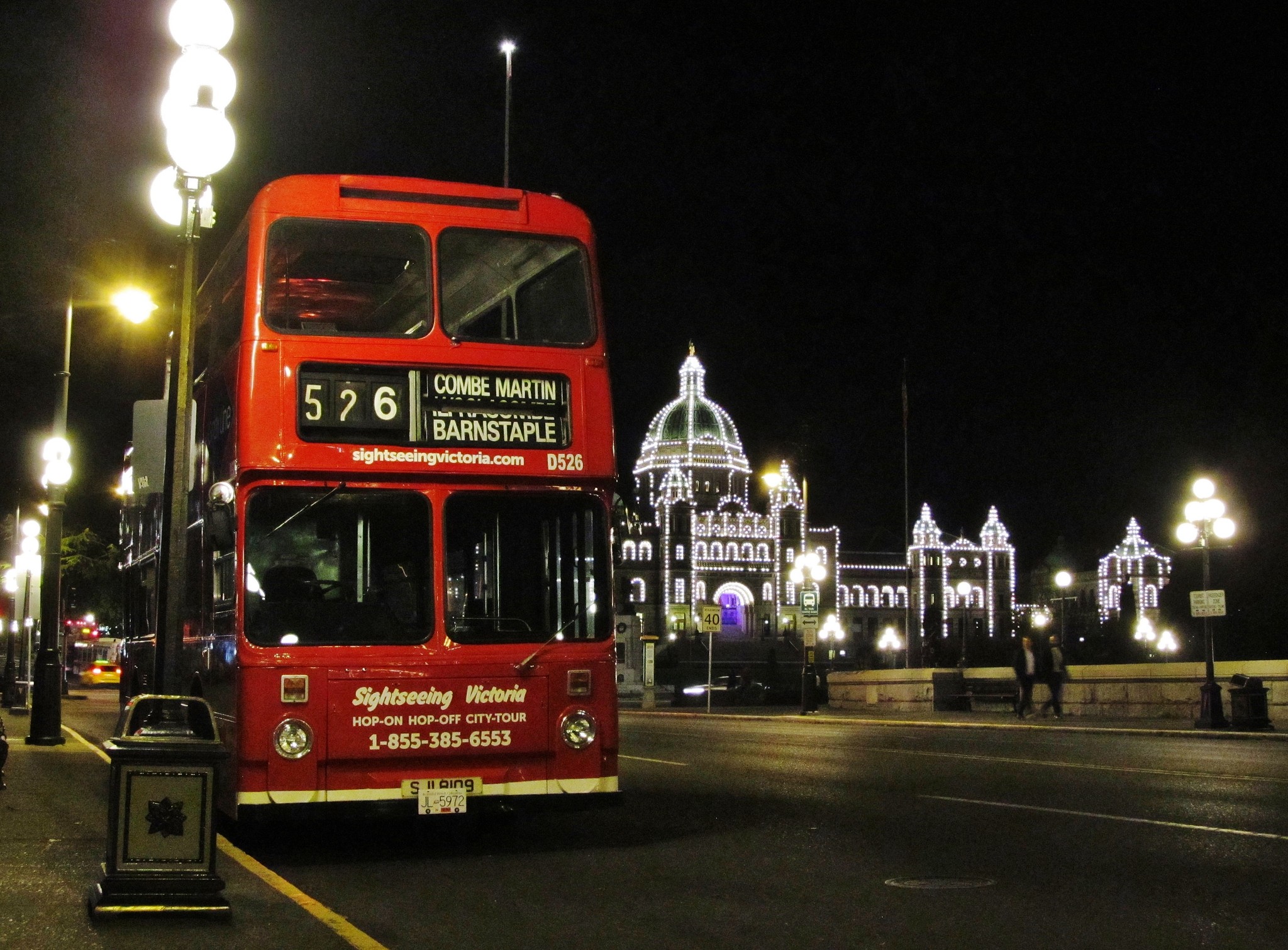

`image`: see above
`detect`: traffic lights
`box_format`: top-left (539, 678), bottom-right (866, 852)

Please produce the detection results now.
top-left (65, 619), bottom-right (73, 626)
top-left (75, 621), bottom-right (86, 626)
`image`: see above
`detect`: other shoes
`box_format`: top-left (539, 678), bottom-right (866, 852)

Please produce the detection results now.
top-left (1016, 713), bottom-right (1036, 721)
top-left (1039, 709), bottom-right (1068, 722)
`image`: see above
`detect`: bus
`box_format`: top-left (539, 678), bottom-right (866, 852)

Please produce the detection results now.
top-left (804, 593), bottom-right (815, 607)
top-left (73, 637), bottom-right (128, 680)
top-left (118, 172), bottom-right (624, 820)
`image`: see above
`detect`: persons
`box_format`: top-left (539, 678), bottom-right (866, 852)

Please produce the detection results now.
top-left (1009, 635), bottom-right (1042, 724)
top-left (1035, 633), bottom-right (1070, 722)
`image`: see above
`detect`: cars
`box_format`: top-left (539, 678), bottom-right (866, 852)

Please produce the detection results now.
top-left (78, 659), bottom-right (121, 688)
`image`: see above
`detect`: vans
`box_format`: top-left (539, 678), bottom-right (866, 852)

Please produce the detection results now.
top-left (34, 617), bottom-right (80, 657)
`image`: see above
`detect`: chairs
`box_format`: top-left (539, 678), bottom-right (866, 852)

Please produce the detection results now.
top-left (261, 565), bottom-right (327, 614)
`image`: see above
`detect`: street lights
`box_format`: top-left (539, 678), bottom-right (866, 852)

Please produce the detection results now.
top-left (1134, 617), bottom-right (1156, 662)
top-left (878, 626), bottom-right (903, 669)
top-left (0, 282), bottom-right (154, 745)
top-left (1175, 475), bottom-right (1240, 730)
top-left (85, 0), bottom-right (241, 928)
top-left (499, 36), bottom-right (518, 188)
top-left (1158, 630), bottom-right (1177, 663)
top-left (762, 449), bottom-right (782, 643)
top-left (819, 614), bottom-right (845, 672)
top-left (1033, 614), bottom-right (1048, 649)
top-left (790, 551), bottom-right (826, 715)
top-left (1055, 571), bottom-right (1070, 648)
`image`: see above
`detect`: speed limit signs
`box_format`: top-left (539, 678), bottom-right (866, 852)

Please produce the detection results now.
top-left (702, 605), bottom-right (722, 633)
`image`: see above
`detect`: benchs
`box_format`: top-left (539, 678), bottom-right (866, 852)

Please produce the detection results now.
top-left (946, 678), bottom-right (1020, 717)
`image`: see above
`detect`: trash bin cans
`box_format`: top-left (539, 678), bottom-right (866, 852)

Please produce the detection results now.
top-left (78, 692), bottom-right (237, 932)
top-left (1228, 673), bottom-right (1274, 732)
